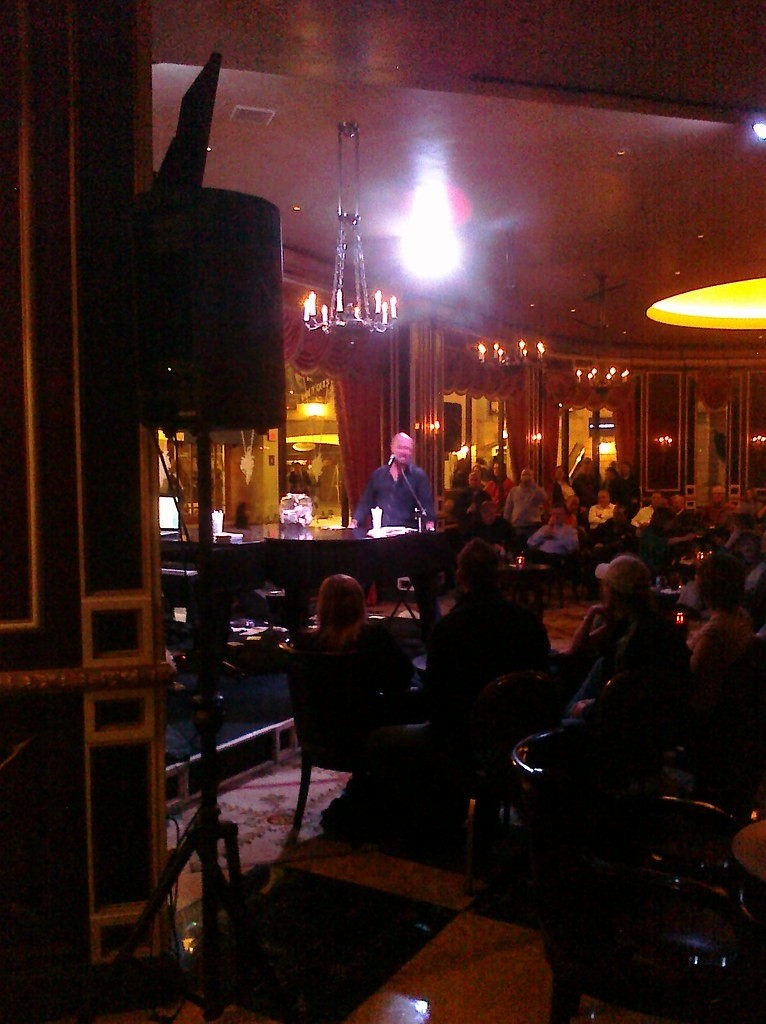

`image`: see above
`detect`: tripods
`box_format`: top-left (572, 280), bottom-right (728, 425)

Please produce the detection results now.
top-left (75, 431), bottom-right (303, 1024)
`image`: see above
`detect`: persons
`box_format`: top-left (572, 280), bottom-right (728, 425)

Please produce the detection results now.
top-left (286, 457), bottom-right (766, 952)
top-left (347, 431), bottom-right (439, 628)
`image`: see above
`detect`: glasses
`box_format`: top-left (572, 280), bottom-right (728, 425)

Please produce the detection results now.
top-left (711, 491), bottom-right (725, 495)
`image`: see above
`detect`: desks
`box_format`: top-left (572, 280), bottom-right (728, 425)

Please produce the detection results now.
top-left (494, 563), bottom-right (550, 624)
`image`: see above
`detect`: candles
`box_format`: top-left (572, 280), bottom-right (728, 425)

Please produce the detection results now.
top-left (303, 287), bottom-right (398, 326)
top-left (574, 367), bottom-right (630, 382)
top-left (477, 339), bottom-right (546, 364)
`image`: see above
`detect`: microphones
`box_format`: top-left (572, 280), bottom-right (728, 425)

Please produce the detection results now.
top-left (388, 455), bottom-right (396, 466)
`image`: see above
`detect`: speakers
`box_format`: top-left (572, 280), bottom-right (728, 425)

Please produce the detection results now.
top-left (130, 185), bottom-right (287, 434)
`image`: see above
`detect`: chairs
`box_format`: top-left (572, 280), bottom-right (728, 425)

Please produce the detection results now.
top-left (268, 620), bottom-right (766, 1023)
top-left (521, 521), bottom-right (586, 609)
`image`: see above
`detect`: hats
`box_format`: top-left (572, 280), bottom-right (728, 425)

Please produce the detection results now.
top-left (695, 553), bottom-right (747, 589)
top-left (594, 554), bottom-right (652, 594)
